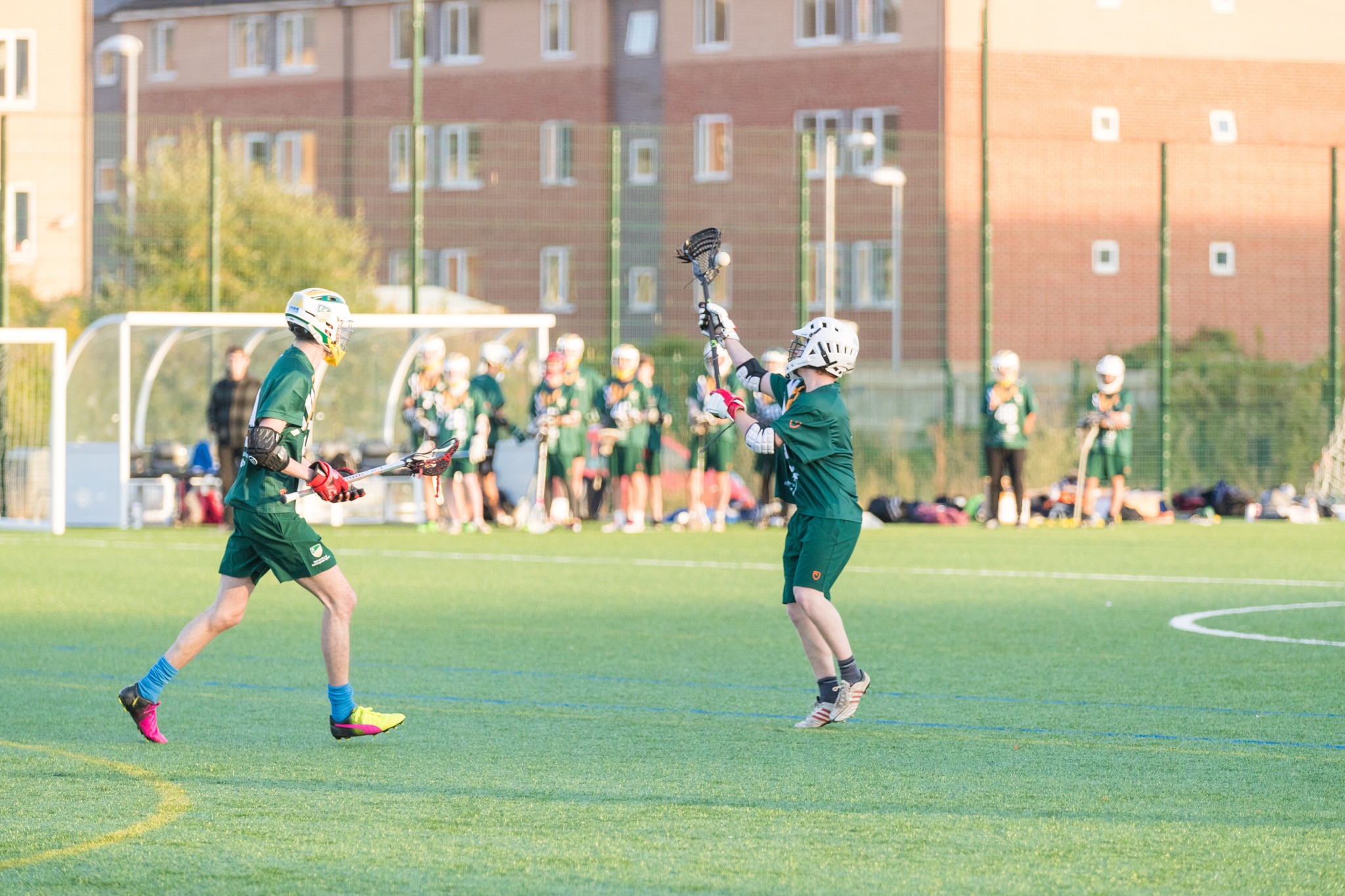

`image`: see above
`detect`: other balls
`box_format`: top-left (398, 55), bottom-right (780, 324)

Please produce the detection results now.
top-left (714, 251), bottom-right (730, 266)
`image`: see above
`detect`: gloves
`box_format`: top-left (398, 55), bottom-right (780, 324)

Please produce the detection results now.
top-left (330, 466), bottom-right (366, 503)
top-left (306, 460), bottom-right (348, 501)
top-left (697, 301), bottom-right (740, 349)
top-left (706, 388), bottom-right (747, 423)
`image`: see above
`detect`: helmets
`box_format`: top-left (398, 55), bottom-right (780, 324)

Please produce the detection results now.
top-left (611, 343), bottom-right (640, 380)
top-left (1095, 354), bottom-right (1125, 394)
top-left (761, 349), bottom-right (781, 374)
top-left (443, 352), bottom-right (471, 387)
top-left (556, 333), bottom-right (585, 372)
top-left (545, 352), bottom-right (567, 374)
top-left (703, 340), bottom-right (733, 377)
top-left (419, 336), bottom-right (446, 371)
top-left (785, 316), bottom-right (860, 379)
top-left (481, 340), bottom-right (512, 374)
top-left (285, 288), bottom-right (354, 366)
top-left (991, 350), bottom-right (1019, 388)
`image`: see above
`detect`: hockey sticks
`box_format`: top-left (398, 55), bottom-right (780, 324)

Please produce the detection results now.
top-left (384, 449), bottom-right (493, 475)
top-left (524, 436), bottom-right (553, 533)
top-left (1074, 426), bottom-right (1100, 520)
top-left (496, 342), bottom-right (524, 385)
top-left (686, 434), bottom-right (712, 532)
top-left (681, 227), bottom-right (722, 389)
top-left (279, 437), bottom-right (460, 504)
top-left (610, 448), bottom-right (626, 531)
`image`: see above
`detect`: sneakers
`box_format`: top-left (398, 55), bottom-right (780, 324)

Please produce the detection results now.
top-left (328, 706), bottom-right (407, 741)
top-left (794, 696), bottom-right (834, 727)
top-left (118, 682), bottom-right (167, 743)
top-left (829, 670), bottom-right (870, 722)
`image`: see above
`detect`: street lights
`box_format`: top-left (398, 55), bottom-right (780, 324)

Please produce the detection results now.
top-left (868, 165), bottom-right (905, 372)
top-left (822, 130), bottom-right (877, 318)
top-left (98, 32), bottom-right (145, 237)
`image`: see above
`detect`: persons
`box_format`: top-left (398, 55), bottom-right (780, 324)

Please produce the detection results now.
top-left (1080, 355), bottom-right (1135, 525)
top-left (117, 288), bottom-right (407, 742)
top-left (402, 330), bottom-right (788, 533)
top-left (696, 301), bottom-right (872, 727)
top-left (980, 349), bottom-right (1037, 528)
top-left (206, 345), bottom-right (264, 530)
